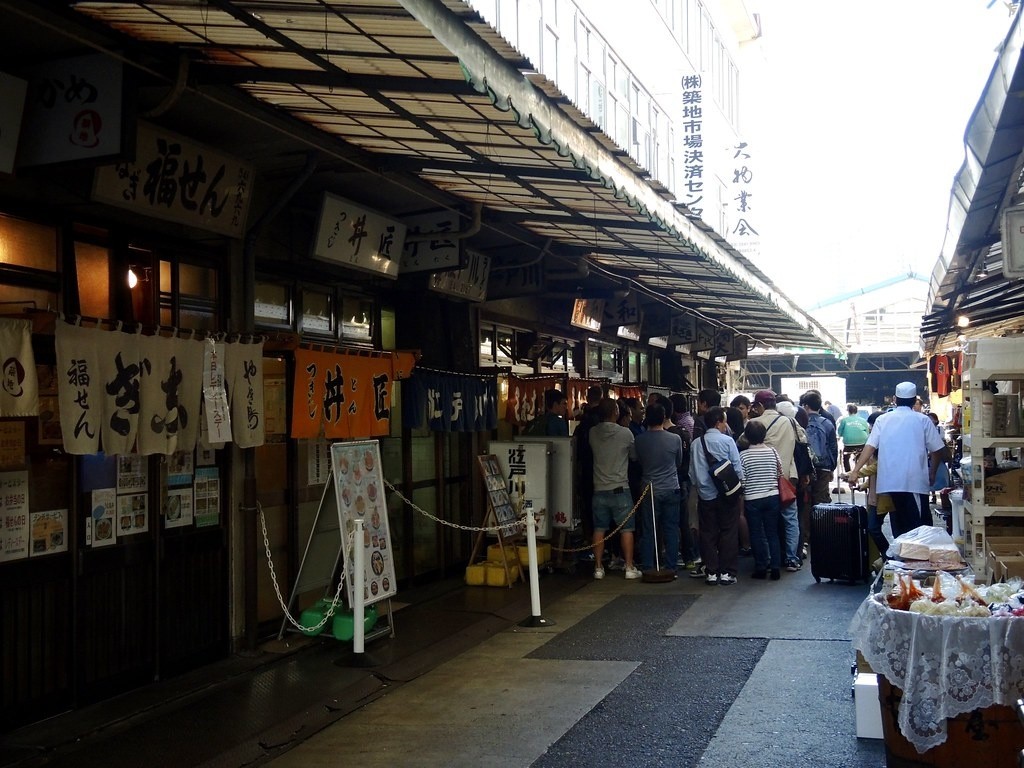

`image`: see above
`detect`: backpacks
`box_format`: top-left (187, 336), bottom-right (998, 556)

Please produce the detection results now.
top-left (803, 415), bottom-right (828, 466)
top-left (666, 425), bottom-right (691, 482)
top-left (521, 411), bottom-right (555, 436)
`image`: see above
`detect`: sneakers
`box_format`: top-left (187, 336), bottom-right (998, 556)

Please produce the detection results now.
top-left (720, 573), bottom-right (738, 585)
top-left (684, 559), bottom-right (696, 568)
top-left (622, 562), bottom-right (640, 571)
top-left (625, 566), bottom-right (643, 579)
top-left (689, 564), bottom-right (707, 577)
top-left (608, 560), bottom-right (623, 570)
top-left (704, 571), bottom-right (718, 585)
top-left (786, 558), bottom-right (801, 571)
top-left (594, 565), bottom-right (605, 579)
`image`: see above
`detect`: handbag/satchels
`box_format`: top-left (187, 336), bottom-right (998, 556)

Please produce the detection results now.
top-left (700, 434), bottom-right (743, 502)
top-left (778, 472), bottom-right (797, 508)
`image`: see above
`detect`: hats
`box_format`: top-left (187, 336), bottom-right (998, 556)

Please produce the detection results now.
top-left (776, 401), bottom-right (798, 419)
top-left (751, 389), bottom-right (775, 405)
top-left (895, 380), bottom-right (916, 399)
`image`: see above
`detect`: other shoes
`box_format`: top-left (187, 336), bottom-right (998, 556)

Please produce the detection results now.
top-left (770, 567), bottom-right (781, 580)
top-left (751, 567), bottom-right (767, 580)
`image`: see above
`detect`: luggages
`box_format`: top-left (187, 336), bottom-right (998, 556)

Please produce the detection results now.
top-left (849, 485), bottom-right (879, 572)
top-left (808, 473), bottom-right (872, 586)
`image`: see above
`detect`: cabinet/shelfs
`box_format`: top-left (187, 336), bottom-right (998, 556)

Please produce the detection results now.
top-left (957, 337), bottom-right (1024, 585)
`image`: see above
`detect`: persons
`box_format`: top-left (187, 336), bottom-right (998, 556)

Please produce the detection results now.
top-left (523, 383), bottom-right (844, 587)
top-left (836, 403), bottom-right (871, 489)
top-left (892, 395), bottom-right (924, 413)
top-left (840, 410), bottom-right (899, 575)
top-left (927, 413), bottom-right (951, 504)
top-left (848, 380), bottom-right (945, 540)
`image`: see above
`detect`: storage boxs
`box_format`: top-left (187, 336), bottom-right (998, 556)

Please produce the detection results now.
top-left (866, 591), bottom-right (1024, 764)
top-left (988, 549), bottom-right (1023, 582)
top-left (983, 533), bottom-right (1024, 574)
top-left (969, 339), bottom-right (1024, 372)
top-left (961, 463), bottom-right (1023, 505)
top-left (466, 559), bottom-right (517, 587)
top-left (485, 540), bottom-right (552, 566)
